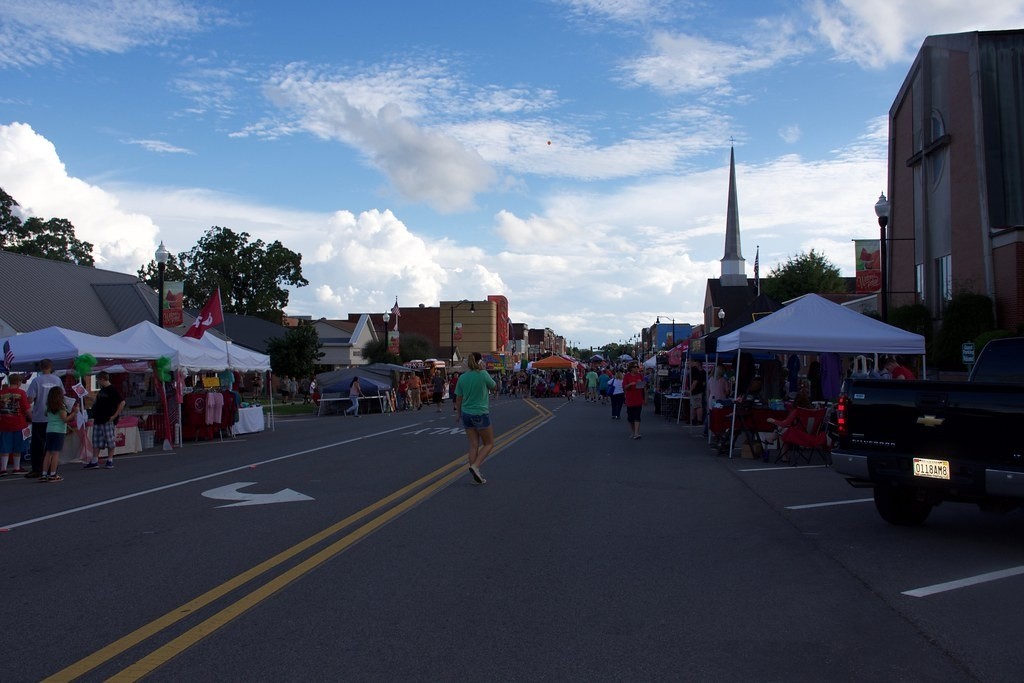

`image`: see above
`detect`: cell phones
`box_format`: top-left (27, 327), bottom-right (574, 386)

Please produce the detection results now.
top-left (478, 359), bottom-right (482, 366)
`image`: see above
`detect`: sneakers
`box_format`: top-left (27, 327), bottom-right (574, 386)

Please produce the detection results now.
top-left (84, 461), bottom-right (100, 469)
top-left (104, 460), bottom-right (114, 468)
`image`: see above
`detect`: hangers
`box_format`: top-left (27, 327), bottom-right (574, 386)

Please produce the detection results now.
top-left (187, 387), bottom-right (234, 398)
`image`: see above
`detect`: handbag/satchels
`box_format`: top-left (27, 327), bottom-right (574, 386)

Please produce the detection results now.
top-left (128, 385), bottom-right (144, 407)
top-left (143, 376), bottom-right (159, 402)
top-left (606, 378), bottom-right (615, 395)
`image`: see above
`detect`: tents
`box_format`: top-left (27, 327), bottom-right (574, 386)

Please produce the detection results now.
top-left (688, 335), bottom-right (779, 426)
top-left (108, 320), bottom-right (230, 447)
top-left (706, 293), bottom-right (786, 440)
top-left (527, 355), bottom-right (576, 398)
top-left (200, 330), bottom-right (276, 432)
top-left (643, 355), bottom-right (657, 368)
top-left (0, 326), bottom-right (172, 464)
top-left (357, 362), bottom-right (422, 379)
top-left (317, 374), bottom-right (393, 417)
top-left (656, 352), bottom-right (689, 423)
top-left (709, 293), bottom-right (926, 459)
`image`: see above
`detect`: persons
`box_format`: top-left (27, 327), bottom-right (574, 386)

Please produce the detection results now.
top-left (456, 352), bottom-right (496, 485)
top-left (397, 355), bottom-right (929, 462)
top-left (344, 376), bottom-right (366, 417)
top-left (0, 360), bottom-right (319, 482)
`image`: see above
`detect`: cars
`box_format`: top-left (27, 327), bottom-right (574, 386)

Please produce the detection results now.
top-left (1, 372), bottom-right (38, 392)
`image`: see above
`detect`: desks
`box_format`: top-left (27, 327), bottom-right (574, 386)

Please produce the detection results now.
top-left (664, 394), bottom-right (690, 423)
top-left (709, 406), bottom-right (787, 456)
top-left (231, 405), bottom-right (265, 435)
top-left (56, 417), bottom-right (143, 460)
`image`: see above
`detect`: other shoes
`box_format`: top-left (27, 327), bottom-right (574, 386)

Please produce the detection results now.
top-left (630, 434), bottom-right (634, 437)
top-left (469, 466), bottom-right (483, 483)
top-left (252, 397), bottom-right (310, 405)
top-left (686, 419), bottom-right (695, 424)
top-left (696, 422), bottom-right (702, 425)
top-left (355, 415), bottom-right (359, 417)
top-left (417, 402), bottom-right (456, 412)
top-left (704, 434), bottom-right (707, 438)
top-left (38, 473), bottom-right (48, 481)
top-left (343, 410), bottom-right (346, 417)
top-left (49, 472), bottom-right (64, 482)
top-left (12, 468), bottom-right (28, 474)
top-left (633, 435), bottom-right (641, 439)
top-left (471, 479), bottom-right (486, 484)
top-left (0, 469), bottom-right (8, 476)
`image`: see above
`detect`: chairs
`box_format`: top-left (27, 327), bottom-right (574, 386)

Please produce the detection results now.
top-left (776, 409), bottom-right (826, 465)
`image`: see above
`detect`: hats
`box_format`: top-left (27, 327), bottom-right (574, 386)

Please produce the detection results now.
top-left (436, 370), bottom-right (441, 373)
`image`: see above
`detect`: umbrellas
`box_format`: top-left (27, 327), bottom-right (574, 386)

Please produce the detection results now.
top-left (618, 354), bottom-right (633, 361)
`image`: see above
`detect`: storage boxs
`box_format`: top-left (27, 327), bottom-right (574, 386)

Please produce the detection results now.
top-left (742, 443), bottom-right (761, 459)
top-left (768, 402), bottom-right (785, 409)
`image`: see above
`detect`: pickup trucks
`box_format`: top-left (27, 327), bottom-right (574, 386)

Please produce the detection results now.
top-left (829, 338), bottom-right (1024, 521)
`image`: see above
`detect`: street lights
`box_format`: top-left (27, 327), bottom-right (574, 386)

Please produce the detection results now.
top-left (656, 316), bottom-right (674, 348)
top-left (383, 310), bottom-right (390, 351)
top-left (638, 333), bottom-right (644, 360)
top-left (718, 308), bottom-right (725, 327)
top-left (155, 240), bottom-right (169, 327)
top-left (630, 337), bottom-right (636, 349)
top-left (875, 192), bottom-right (891, 324)
top-left (451, 300), bottom-right (475, 367)
top-left (634, 334), bottom-right (638, 350)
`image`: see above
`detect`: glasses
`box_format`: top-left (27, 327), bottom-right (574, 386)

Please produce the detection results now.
top-left (633, 366), bottom-right (639, 368)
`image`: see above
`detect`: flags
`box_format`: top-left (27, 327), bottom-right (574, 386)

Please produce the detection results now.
top-left (392, 302), bottom-right (401, 317)
top-left (3, 342), bottom-right (15, 370)
top-left (180, 288), bottom-right (224, 340)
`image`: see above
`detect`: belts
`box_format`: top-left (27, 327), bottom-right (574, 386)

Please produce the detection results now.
top-left (412, 388), bottom-right (418, 389)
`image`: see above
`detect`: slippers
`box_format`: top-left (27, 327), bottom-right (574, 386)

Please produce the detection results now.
top-left (782, 460), bottom-right (787, 463)
top-left (762, 440), bottom-right (774, 445)
